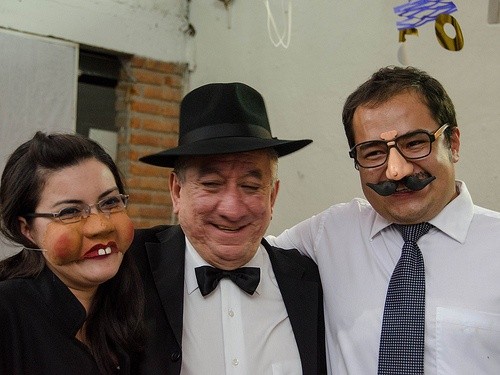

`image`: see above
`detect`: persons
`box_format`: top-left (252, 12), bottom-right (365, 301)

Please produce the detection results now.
top-left (0, 131), bottom-right (145, 375)
top-left (263, 67), bottom-right (500, 375)
top-left (90, 83), bottom-right (327, 375)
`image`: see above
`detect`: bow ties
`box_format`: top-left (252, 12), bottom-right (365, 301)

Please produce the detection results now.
top-left (194, 265), bottom-right (261, 297)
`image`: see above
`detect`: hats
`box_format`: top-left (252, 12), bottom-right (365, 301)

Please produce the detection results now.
top-left (138, 82), bottom-right (313, 169)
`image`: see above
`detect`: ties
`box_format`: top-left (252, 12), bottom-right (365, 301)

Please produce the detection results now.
top-left (377, 222), bottom-right (436, 375)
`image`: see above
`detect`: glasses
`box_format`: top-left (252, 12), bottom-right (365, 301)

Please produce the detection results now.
top-left (29, 193), bottom-right (130, 224)
top-left (348, 123), bottom-right (449, 169)
